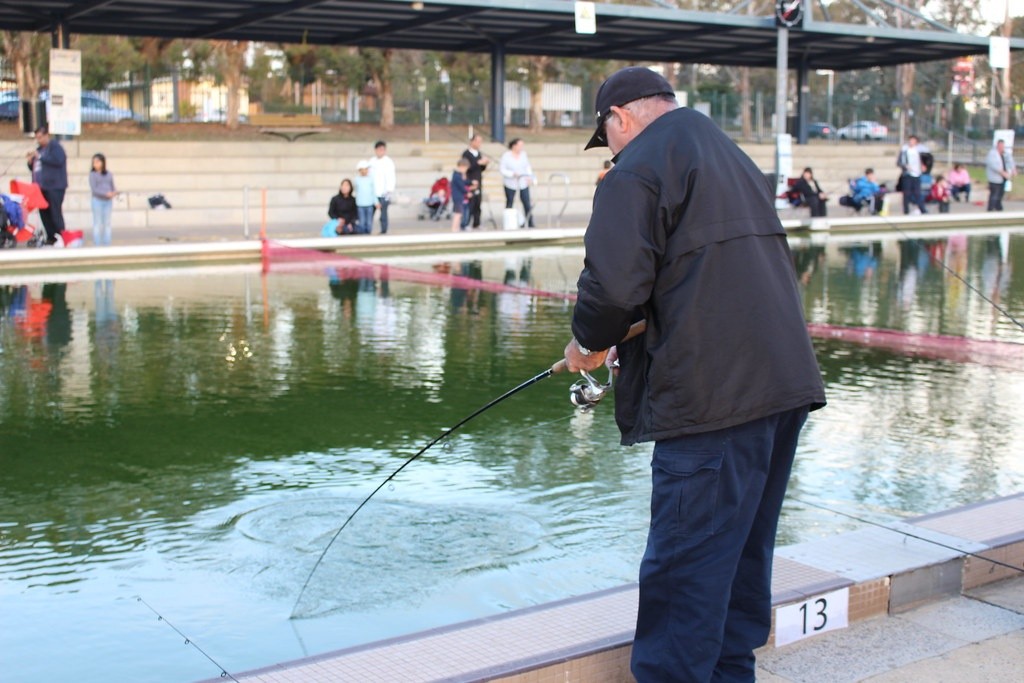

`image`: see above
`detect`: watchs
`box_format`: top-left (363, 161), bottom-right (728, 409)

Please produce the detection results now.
top-left (575, 338), bottom-right (598, 355)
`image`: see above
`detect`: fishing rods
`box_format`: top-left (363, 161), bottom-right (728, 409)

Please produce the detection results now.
top-left (138, 594), bottom-right (239, 683)
top-left (785, 494), bottom-right (1024, 572)
top-left (858, 192), bottom-right (1024, 330)
top-left (288, 358), bottom-right (569, 613)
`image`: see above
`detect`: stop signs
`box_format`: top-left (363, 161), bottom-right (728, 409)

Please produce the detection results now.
top-left (952, 57), bottom-right (971, 74)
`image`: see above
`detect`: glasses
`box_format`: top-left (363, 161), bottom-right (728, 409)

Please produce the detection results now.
top-left (597, 110), bottom-right (615, 142)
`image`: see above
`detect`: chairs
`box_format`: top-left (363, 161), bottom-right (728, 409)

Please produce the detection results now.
top-left (788, 177), bottom-right (828, 207)
top-left (847, 179), bottom-right (888, 210)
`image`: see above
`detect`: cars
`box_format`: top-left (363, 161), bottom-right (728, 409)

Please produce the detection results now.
top-left (807, 121), bottom-right (838, 140)
top-left (836, 120), bottom-right (888, 141)
top-left (0, 86), bottom-right (142, 123)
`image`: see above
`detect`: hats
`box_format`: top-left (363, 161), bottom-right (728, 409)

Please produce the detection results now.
top-left (356, 161), bottom-right (370, 171)
top-left (583, 66), bottom-right (676, 151)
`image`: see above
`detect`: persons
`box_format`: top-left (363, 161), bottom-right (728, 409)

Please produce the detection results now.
top-left (319, 140), bottom-right (395, 233)
top-left (839, 167), bottom-right (889, 215)
top-left (38, 282), bottom-right (78, 381)
top-left (787, 167), bottom-right (830, 217)
top-left (836, 241), bottom-right (885, 286)
top-left (424, 135), bottom-right (488, 232)
top-left (88, 153), bottom-right (117, 249)
top-left (430, 258), bottom-right (488, 346)
top-left (789, 247), bottom-right (826, 290)
top-left (982, 236), bottom-right (1010, 307)
top-left (26, 125), bottom-right (68, 246)
top-left (595, 159), bottom-right (613, 185)
top-left (496, 254), bottom-right (532, 352)
top-left (569, 66), bottom-right (827, 683)
top-left (987, 140), bottom-right (1016, 212)
top-left (499, 139), bottom-right (535, 229)
top-left (325, 263), bottom-right (395, 366)
top-left (896, 135), bottom-right (972, 215)
top-left (94, 278), bottom-right (119, 387)
top-left (894, 234), bottom-right (971, 313)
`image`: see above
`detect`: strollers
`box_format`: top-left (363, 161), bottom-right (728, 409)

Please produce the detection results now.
top-left (416, 176), bottom-right (455, 221)
top-left (2, 179), bottom-right (49, 248)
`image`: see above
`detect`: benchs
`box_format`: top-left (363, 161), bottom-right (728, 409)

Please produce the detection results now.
top-left (250, 113), bottom-right (332, 141)
